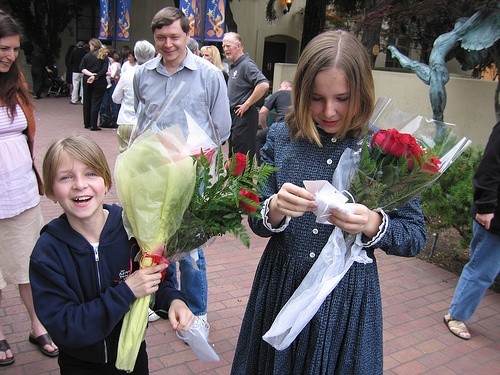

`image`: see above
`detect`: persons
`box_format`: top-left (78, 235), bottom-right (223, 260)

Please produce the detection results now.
top-left (119, 50), bottom-right (138, 77)
top-left (101, 45), bottom-right (121, 128)
top-left (132, 7), bottom-right (232, 337)
top-left (186, 38), bottom-right (200, 56)
top-left (0, 10), bottom-right (58, 367)
top-left (29, 135), bottom-right (195, 375)
top-left (222, 32), bottom-right (269, 164)
top-left (69, 41), bottom-right (88, 104)
top-left (80, 38), bottom-right (109, 131)
top-left (200, 45), bottom-right (229, 86)
top-left (111, 40), bottom-right (156, 154)
top-left (65, 41), bottom-right (76, 97)
top-left (254, 79), bottom-right (292, 167)
top-left (387, 0), bottom-right (500, 143)
top-left (443, 121), bottom-right (500, 339)
top-left (106, 53), bottom-right (121, 89)
top-left (229, 29), bottom-right (429, 375)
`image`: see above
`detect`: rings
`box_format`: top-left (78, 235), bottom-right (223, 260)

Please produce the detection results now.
top-left (482, 224), bottom-right (485, 226)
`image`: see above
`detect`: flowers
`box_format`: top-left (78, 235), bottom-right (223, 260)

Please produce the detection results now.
top-left (150, 134), bottom-right (282, 257)
top-left (339, 121), bottom-right (443, 238)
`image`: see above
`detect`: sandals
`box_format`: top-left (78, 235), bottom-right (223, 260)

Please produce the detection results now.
top-left (444, 313), bottom-right (471, 340)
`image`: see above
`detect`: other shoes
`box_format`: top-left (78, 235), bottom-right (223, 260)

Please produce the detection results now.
top-left (84, 125), bottom-right (102, 131)
top-left (148, 307), bottom-right (160, 322)
top-left (184, 313), bottom-right (210, 345)
top-left (31, 92), bottom-right (41, 99)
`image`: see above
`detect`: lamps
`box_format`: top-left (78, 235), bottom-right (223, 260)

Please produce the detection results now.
top-left (283, 0), bottom-right (293, 14)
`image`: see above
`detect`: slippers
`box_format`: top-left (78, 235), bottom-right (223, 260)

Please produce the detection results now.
top-left (29, 332), bottom-right (59, 358)
top-left (0, 339), bottom-right (15, 365)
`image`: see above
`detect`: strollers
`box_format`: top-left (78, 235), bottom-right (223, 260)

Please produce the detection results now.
top-left (44, 65), bottom-right (70, 99)
